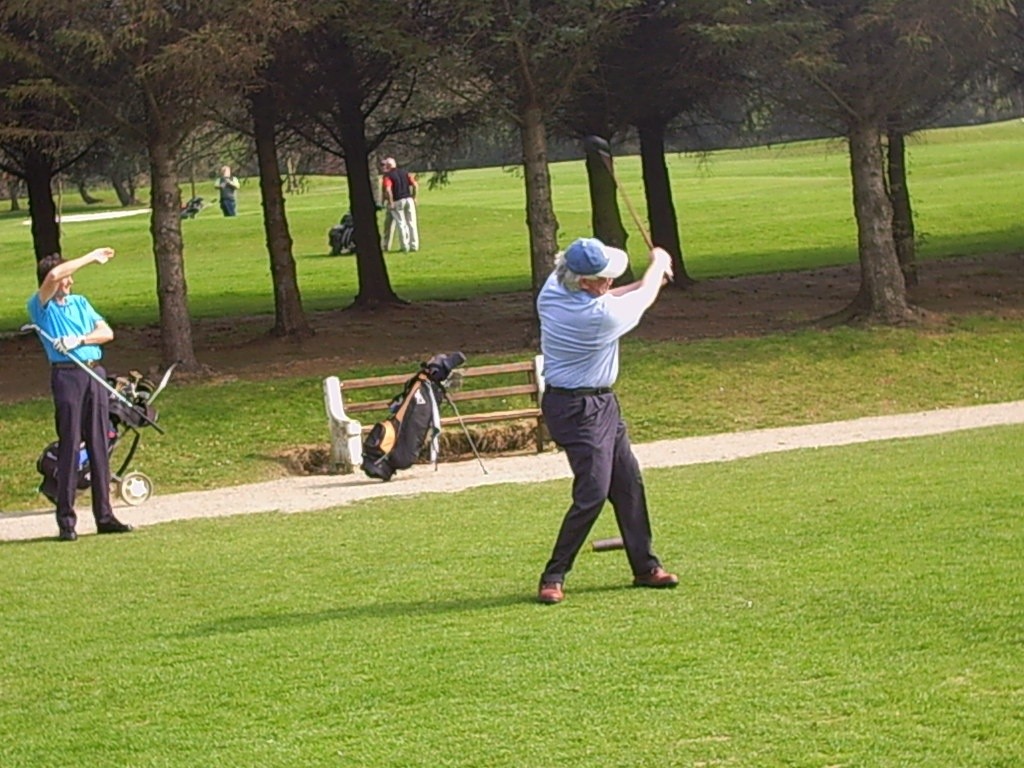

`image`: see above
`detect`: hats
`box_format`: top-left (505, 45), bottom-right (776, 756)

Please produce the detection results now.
top-left (564, 238), bottom-right (628, 278)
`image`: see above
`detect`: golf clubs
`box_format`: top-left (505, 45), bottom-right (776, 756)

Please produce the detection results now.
top-left (21, 322), bottom-right (166, 436)
top-left (590, 136), bottom-right (678, 286)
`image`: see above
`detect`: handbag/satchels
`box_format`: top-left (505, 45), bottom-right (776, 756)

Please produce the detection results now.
top-left (37, 441), bottom-right (60, 479)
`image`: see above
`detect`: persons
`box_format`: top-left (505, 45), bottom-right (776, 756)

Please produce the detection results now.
top-left (215, 165), bottom-right (240, 216)
top-left (24, 248), bottom-right (134, 541)
top-left (536, 238), bottom-right (679, 604)
top-left (378, 157), bottom-right (420, 253)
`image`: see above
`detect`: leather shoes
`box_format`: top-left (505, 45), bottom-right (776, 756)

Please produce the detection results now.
top-left (633, 566), bottom-right (679, 585)
top-left (98, 517), bottom-right (132, 531)
top-left (539, 582), bottom-right (563, 602)
top-left (60, 527), bottom-right (77, 540)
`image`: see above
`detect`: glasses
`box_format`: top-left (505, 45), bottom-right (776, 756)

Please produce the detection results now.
top-left (380, 163), bottom-right (385, 167)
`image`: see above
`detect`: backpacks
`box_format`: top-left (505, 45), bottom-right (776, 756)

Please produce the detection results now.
top-left (361, 416), bottom-right (401, 458)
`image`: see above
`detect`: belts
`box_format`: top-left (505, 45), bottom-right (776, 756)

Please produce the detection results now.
top-left (545, 386), bottom-right (613, 395)
top-left (52, 359), bottom-right (99, 369)
